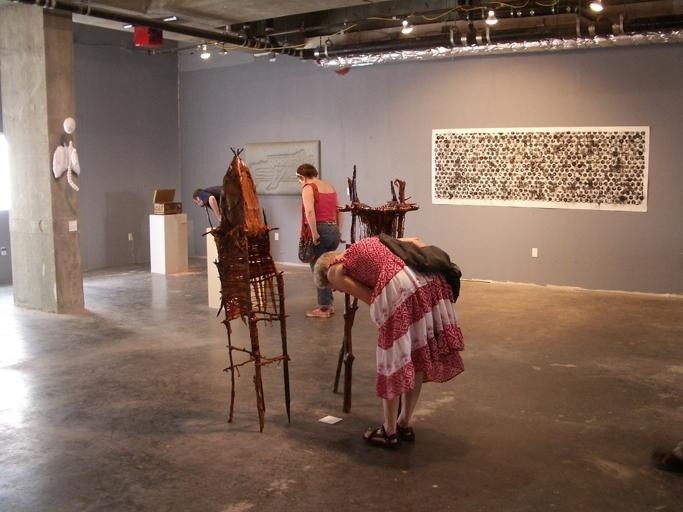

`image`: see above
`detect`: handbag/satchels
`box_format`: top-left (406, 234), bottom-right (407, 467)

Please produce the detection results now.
top-left (298, 224), bottom-right (315, 264)
top-left (377, 232), bottom-right (461, 302)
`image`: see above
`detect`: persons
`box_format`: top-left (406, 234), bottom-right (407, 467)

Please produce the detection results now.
top-left (295, 163), bottom-right (347, 320)
top-left (191, 186), bottom-right (224, 223)
top-left (313, 233), bottom-right (466, 448)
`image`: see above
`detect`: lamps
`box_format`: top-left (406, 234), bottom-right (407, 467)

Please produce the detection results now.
top-left (267, 50), bottom-right (278, 62)
top-left (198, 42), bottom-right (229, 59)
top-left (484, 8), bottom-right (499, 26)
top-left (589, 0), bottom-right (604, 12)
top-left (400, 18), bottom-right (413, 34)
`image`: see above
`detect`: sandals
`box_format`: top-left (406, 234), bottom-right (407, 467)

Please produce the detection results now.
top-left (362, 423), bottom-right (416, 451)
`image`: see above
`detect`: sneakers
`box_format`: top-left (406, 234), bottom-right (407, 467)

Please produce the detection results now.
top-left (304, 305), bottom-right (334, 318)
